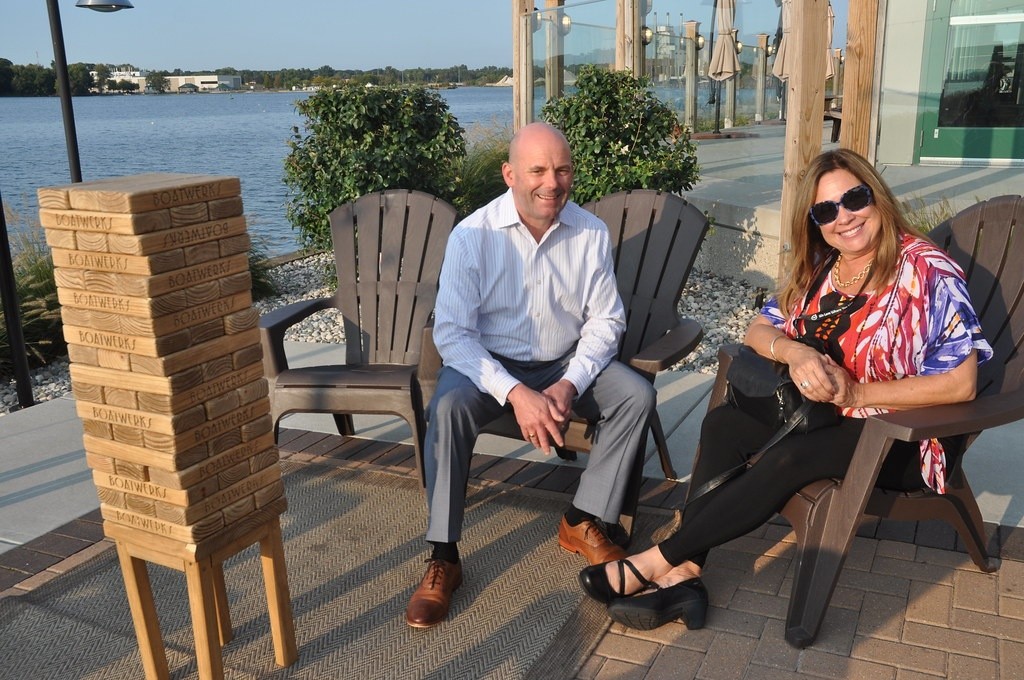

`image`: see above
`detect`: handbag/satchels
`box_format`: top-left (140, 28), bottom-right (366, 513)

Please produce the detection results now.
top-left (722, 337), bottom-right (837, 435)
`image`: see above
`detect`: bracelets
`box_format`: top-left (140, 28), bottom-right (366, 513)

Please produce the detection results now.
top-left (771, 334), bottom-right (790, 365)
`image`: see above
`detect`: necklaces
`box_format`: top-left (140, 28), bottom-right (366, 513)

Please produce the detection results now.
top-left (834, 253), bottom-right (875, 286)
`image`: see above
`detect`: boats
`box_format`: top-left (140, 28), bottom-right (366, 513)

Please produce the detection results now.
top-left (447, 86), bottom-right (458, 89)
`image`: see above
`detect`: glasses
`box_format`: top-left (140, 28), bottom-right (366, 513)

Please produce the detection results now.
top-left (809, 185), bottom-right (872, 225)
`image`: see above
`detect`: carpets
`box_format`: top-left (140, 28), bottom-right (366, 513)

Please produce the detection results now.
top-left (0, 460), bottom-right (680, 680)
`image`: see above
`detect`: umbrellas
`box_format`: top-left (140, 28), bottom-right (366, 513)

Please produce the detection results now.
top-left (771, 0), bottom-right (835, 119)
top-left (707, 0), bottom-right (740, 133)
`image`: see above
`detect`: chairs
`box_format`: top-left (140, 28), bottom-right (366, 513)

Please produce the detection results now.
top-left (486, 188), bottom-right (709, 549)
top-left (253, 186), bottom-right (455, 489)
top-left (692, 195), bottom-right (1024, 650)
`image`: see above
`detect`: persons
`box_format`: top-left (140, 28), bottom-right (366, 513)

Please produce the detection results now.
top-left (579, 148), bottom-right (995, 631)
top-left (406, 121), bottom-right (657, 628)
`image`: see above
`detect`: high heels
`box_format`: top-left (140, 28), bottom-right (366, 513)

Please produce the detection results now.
top-left (577, 558), bottom-right (653, 603)
top-left (606, 577), bottom-right (708, 630)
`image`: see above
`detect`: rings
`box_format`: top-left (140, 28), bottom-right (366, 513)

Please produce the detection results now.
top-left (801, 381), bottom-right (810, 388)
top-left (529, 434), bottom-right (537, 437)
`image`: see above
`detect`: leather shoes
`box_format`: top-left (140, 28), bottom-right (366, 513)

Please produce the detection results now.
top-left (404, 558), bottom-right (463, 628)
top-left (558, 513), bottom-right (628, 565)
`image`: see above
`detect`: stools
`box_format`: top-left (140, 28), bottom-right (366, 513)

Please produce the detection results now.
top-left (103, 496), bottom-right (301, 680)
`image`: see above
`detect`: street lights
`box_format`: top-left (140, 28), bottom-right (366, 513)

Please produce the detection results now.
top-left (47, 0), bottom-right (134, 183)
